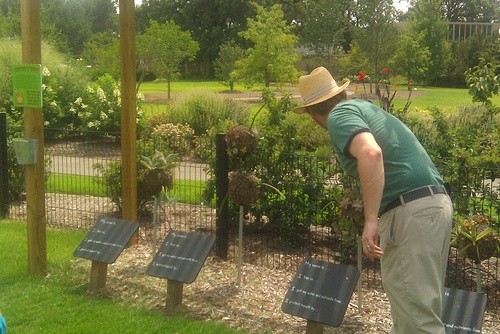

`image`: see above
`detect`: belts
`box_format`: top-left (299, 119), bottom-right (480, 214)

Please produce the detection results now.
top-left (377, 186), bottom-right (445, 219)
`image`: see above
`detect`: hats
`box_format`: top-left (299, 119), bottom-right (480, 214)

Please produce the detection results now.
top-left (291, 65), bottom-right (350, 115)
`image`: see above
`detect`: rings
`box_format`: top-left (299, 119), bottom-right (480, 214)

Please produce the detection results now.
top-left (366, 245), bottom-right (370, 248)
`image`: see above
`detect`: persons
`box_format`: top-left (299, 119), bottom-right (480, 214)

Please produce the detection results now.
top-left (292, 66), bottom-right (453, 333)
